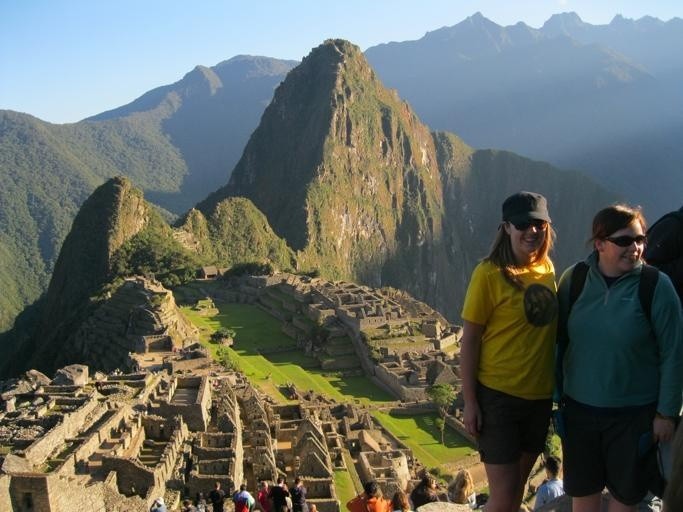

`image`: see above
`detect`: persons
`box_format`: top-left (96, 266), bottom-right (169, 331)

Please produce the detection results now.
top-left (149, 476), bottom-right (317, 512)
top-left (447, 469), bottom-right (477, 511)
top-left (459, 190), bottom-right (562, 512)
top-left (388, 491), bottom-right (415, 512)
top-left (409, 474), bottom-right (440, 510)
top-left (532, 453), bottom-right (565, 511)
top-left (345, 481), bottom-right (394, 511)
top-left (546, 203), bottom-right (682, 511)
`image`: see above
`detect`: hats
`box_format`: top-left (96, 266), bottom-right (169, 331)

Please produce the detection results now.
top-left (503, 190), bottom-right (549, 223)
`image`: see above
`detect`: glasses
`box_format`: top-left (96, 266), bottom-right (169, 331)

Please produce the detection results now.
top-left (507, 220), bottom-right (549, 231)
top-left (599, 235), bottom-right (647, 247)
